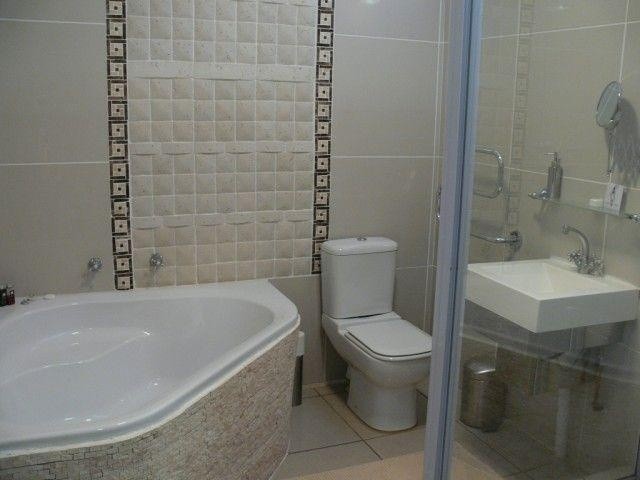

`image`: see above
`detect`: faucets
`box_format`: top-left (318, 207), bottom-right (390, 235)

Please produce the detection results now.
top-left (561, 223), bottom-right (596, 273)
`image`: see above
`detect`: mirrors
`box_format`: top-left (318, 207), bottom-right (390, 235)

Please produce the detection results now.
top-left (596, 81), bottom-right (625, 175)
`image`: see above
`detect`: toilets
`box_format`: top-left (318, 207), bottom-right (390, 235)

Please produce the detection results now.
top-left (320, 237), bottom-right (433, 432)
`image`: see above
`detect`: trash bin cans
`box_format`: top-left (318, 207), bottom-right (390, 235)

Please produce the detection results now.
top-left (459, 355), bottom-right (506, 432)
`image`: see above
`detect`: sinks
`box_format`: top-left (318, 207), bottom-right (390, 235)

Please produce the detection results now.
top-left (466, 256), bottom-right (638, 331)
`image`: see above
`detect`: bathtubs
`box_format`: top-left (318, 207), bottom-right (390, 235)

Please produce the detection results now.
top-left (0, 276), bottom-right (300, 479)
